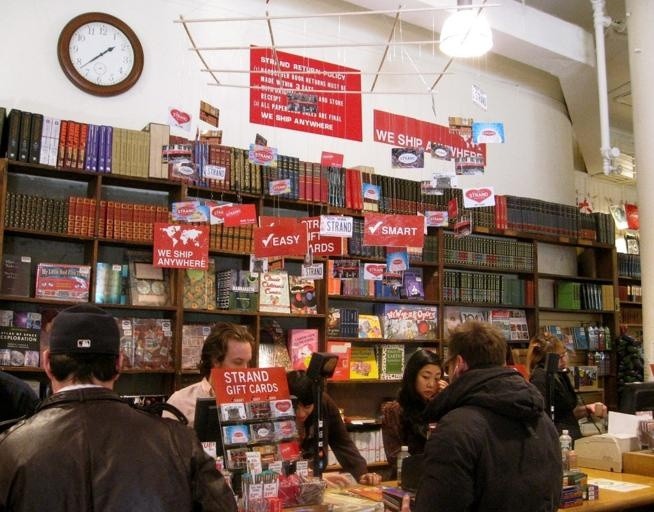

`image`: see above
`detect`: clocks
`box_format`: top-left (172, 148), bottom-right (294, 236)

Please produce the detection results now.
top-left (57, 8), bottom-right (145, 96)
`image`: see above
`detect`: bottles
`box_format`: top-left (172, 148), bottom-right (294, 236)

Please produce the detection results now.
top-left (558, 430), bottom-right (573, 473)
top-left (397, 445), bottom-right (412, 487)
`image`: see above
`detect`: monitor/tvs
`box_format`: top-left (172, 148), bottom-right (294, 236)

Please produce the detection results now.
top-left (618, 381), bottom-right (654, 414)
top-left (193, 397), bottom-right (223, 457)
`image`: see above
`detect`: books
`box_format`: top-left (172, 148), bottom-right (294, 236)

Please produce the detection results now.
top-left (0, 107), bottom-right (642, 387)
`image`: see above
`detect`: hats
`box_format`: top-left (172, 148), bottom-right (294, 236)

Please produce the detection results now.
top-left (48, 305), bottom-right (122, 350)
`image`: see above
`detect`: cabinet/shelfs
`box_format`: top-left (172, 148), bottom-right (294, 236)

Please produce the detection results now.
top-left (0, 110), bottom-right (646, 471)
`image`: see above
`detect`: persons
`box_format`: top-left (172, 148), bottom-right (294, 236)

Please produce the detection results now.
top-left (160, 321), bottom-right (256, 432)
top-left (0, 303), bottom-right (239, 512)
top-left (382, 349), bottom-right (450, 483)
top-left (286, 370), bottom-right (382, 486)
top-left (1, 371), bottom-right (39, 435)
top-left (383, 320), bottom-right (563, 512)
top-left (525, 329), bottom-right (607, 448)
